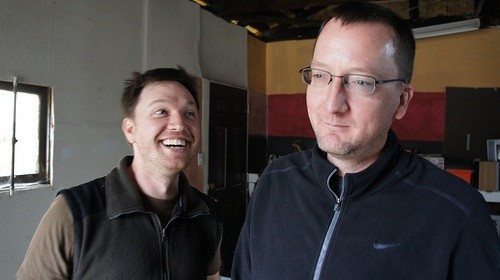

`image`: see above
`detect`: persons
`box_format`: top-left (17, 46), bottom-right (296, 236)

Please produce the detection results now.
top-left (230, 0), bottom-right (500, 280)
top-left (15, 65), bottom-right (223, 280)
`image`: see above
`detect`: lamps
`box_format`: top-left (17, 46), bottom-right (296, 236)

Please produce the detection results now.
top-left (412, 18), bottom-right (481, 39)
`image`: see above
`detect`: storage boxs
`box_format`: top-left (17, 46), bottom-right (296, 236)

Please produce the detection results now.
top-left (445, 168), bottom-right (475, 186)
top-left (478, 161), bottom-right (499, 191)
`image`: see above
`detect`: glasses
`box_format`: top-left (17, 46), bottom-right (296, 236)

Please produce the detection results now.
top-left (298, 66), bottom-right (407, 95)
